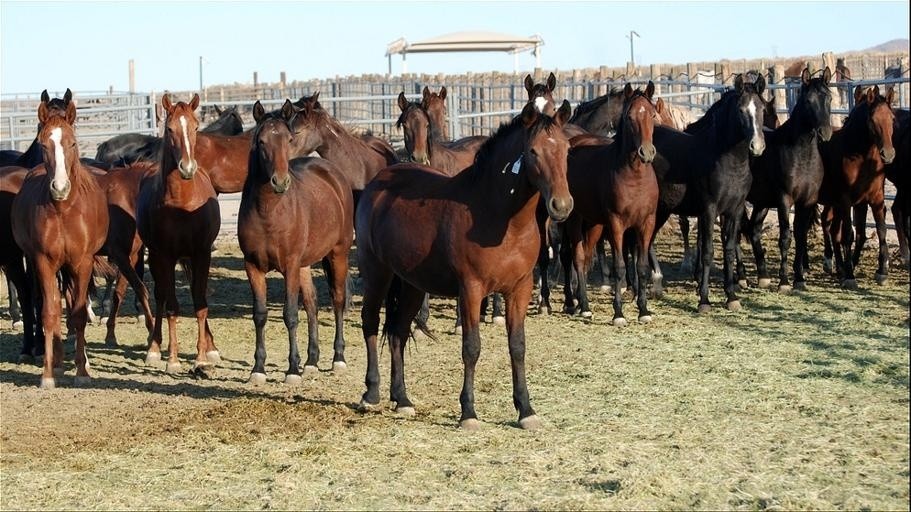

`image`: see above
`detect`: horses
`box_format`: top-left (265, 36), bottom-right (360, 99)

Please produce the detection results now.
top-left (134, 95), bottom-right (223, 372)
top-left (357, 97), bottom-right (574, 430)
top-left (238, 98), bottom-right (355, 385)
top-left (3, 88), bottom-right (281, 357)
top-left (11, 102), bottom-right (109, 390)
top-left (280, 65), bottom-right (910, 325)
